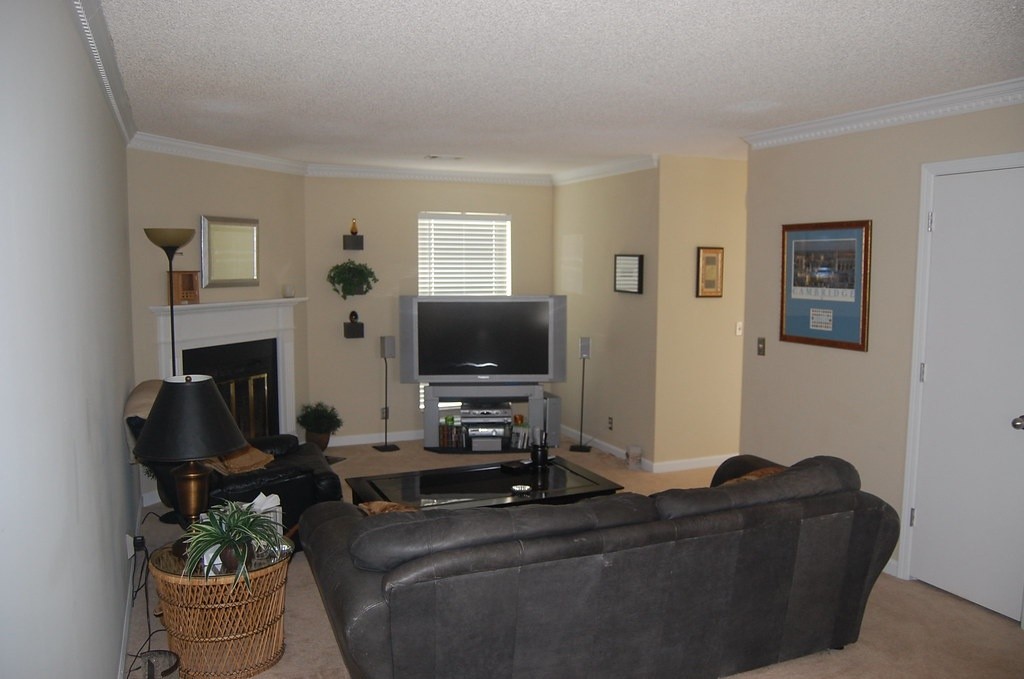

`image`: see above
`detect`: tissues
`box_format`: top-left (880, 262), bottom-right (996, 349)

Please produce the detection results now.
top-left (198, 490), bottom-right (284, 566)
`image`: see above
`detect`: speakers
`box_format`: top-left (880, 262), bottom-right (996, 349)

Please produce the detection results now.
top-left (579, 337), bottom-right (590, 358)
top-left (380, 336), bottom-right (396, 358)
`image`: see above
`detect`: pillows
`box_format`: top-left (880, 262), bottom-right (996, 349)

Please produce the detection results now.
top-left (358, 500), bottom-right (417, 517)
top-left (717, 462), bottom-right (786, 486)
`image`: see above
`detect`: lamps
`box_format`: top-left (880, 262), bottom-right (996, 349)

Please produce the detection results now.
top-left (568, 336), bottom-right (592, 451)
top-left (372, 335), bottom-right (398, 453)
top-left (134, 372), bottom-right (250, 562)
top-left (144, 227), bottom-right (195, 374)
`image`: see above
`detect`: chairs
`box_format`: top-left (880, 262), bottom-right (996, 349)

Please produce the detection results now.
top-left (117, 376), bottom-right (339, 533)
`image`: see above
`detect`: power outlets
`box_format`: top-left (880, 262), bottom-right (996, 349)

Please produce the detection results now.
top-left (607, 416), bottom-right (613, 432)
top-left (754, 335), bottom-right (765, 357)
top-left (380, 406), bottom-right (389, 418)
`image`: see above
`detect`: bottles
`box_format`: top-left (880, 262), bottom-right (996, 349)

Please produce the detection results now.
top-left (349, 311), bottom-right (358, 323)
top-left (282, 280), bottom-right (296, 298)
top-left (351, 219), bottom-right (357, 236)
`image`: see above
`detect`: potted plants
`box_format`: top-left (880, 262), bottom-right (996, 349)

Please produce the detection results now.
top-left (149, 503), bottom-right (292, 678)
top-left (297, 403), bottom-right (342, 454)
top-left (327, 260), bottom-right (380, 298)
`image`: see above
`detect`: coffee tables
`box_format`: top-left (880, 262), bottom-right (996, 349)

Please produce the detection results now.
top-left (345, 455), bottom-right (622, 527)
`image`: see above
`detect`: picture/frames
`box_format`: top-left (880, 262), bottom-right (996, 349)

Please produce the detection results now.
top-left (778, 218), bottom-right (873, 353)
top-left (203, 215), bottom-right (260, 286)
top-left (610, 254), bottom-right (643, 295)
top-left (697, 245), bottom-right (726, 300)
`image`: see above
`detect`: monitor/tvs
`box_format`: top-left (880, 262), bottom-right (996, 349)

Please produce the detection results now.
top-left (398, 295), bottom-right (567, 384)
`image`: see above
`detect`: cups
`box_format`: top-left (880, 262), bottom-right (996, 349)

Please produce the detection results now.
top-left (530, 442), bottom-right (548, 469)
top-left (514, 415), bottom-right (524, 425)
top-left (445, 416), bottom-right (454, 425)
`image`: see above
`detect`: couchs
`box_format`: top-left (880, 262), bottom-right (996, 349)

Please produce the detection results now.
top-left (296, 448), bottom-right (902, 679)
